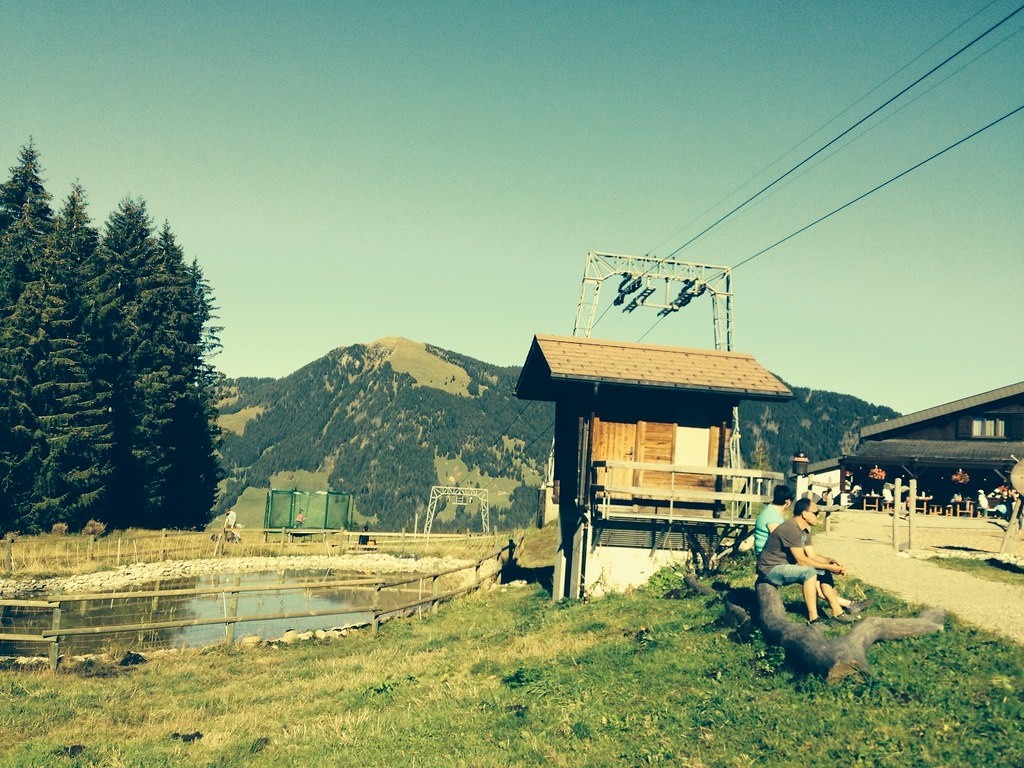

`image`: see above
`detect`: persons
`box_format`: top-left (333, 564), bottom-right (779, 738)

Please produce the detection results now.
top-left (974, 489), bottom-right (988, 518)
top-left (296, 507), bottom-right (307, 528)
top-left (987, 485), bottom-right (1019, 522)
top-left (223, 508), bottom-right (236, 542)
top-left (754, 485), bottom-right (873, 624)
top-left (757, 498), bottom-right (857, 631)
top-left (880, 484), bottom-right (894, 512)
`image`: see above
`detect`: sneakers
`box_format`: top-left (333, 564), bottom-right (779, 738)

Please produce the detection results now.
top-left (806, 619), bottom-right (830, 633)
top-left (836, 614), bottom-right (856, 624)
top-left (848, 599), bottom-right (873, 615)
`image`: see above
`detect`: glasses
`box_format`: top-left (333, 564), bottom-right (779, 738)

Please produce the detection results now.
top-left (806, 509), bottom-right (820, 517)
